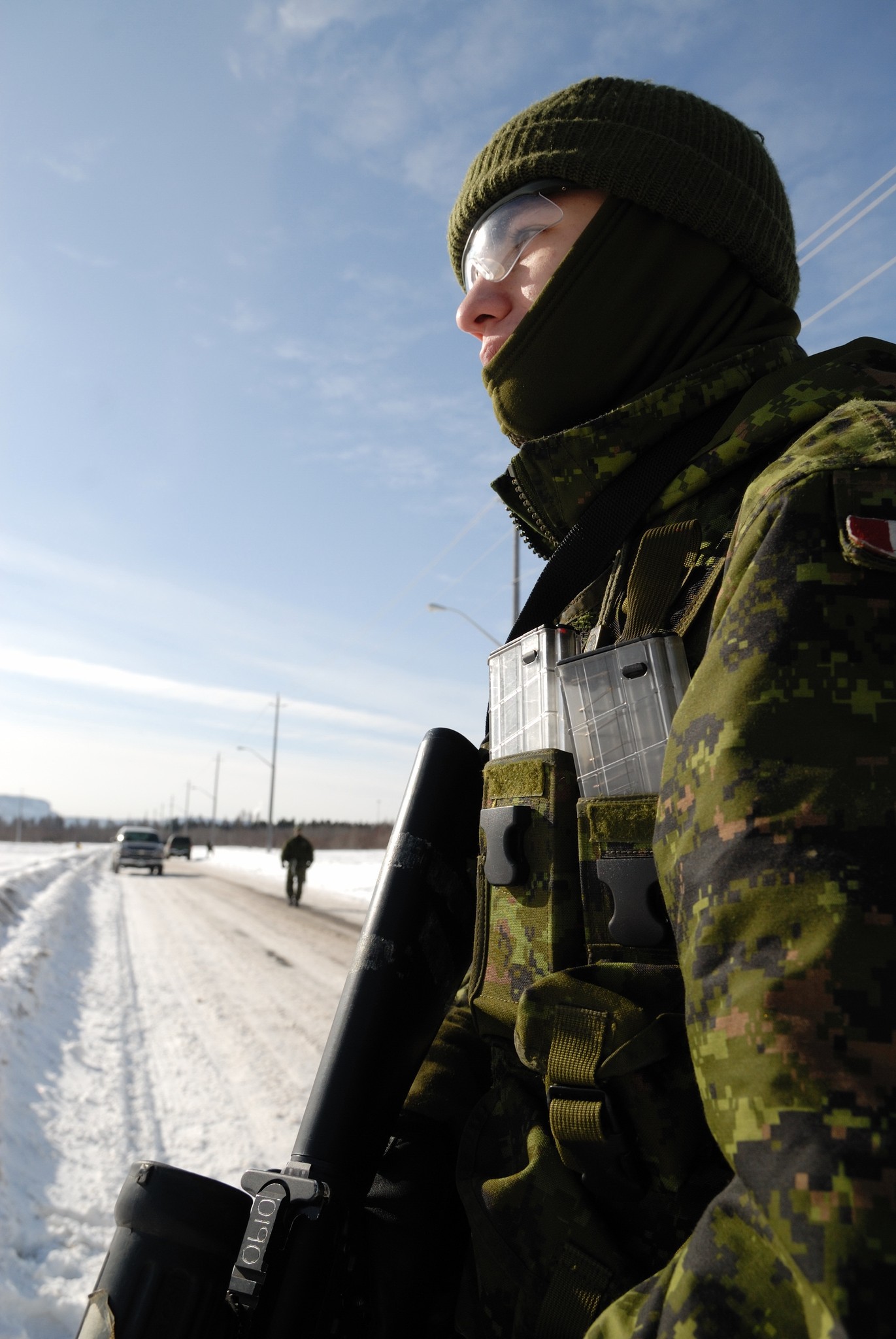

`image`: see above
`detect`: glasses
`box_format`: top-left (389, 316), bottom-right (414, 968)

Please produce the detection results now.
top-left (460, 180), bottom-right (569, 289)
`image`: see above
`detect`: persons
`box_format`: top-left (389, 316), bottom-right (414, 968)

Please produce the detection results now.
top-left (280, 825), bottom-right (314, 906)
top-left (280, 78), bottom-right (894, 1339)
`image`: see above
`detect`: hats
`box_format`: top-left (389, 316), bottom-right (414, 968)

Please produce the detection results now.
top-left (448, 77), bottom-right (802, 308)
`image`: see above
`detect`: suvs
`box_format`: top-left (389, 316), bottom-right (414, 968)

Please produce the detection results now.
top-left (110, 824), bottom-right (165, 875)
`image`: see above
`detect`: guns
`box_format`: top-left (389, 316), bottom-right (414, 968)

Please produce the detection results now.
top-left (69, 722), bottom-right (483, 1338)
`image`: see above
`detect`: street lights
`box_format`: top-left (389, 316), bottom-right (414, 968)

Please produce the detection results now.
top-left (237, 694), bottom-right (281, 854)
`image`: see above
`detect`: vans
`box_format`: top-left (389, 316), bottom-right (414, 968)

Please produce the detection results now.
top-left (164, 835), bottom-right (193, 858)
